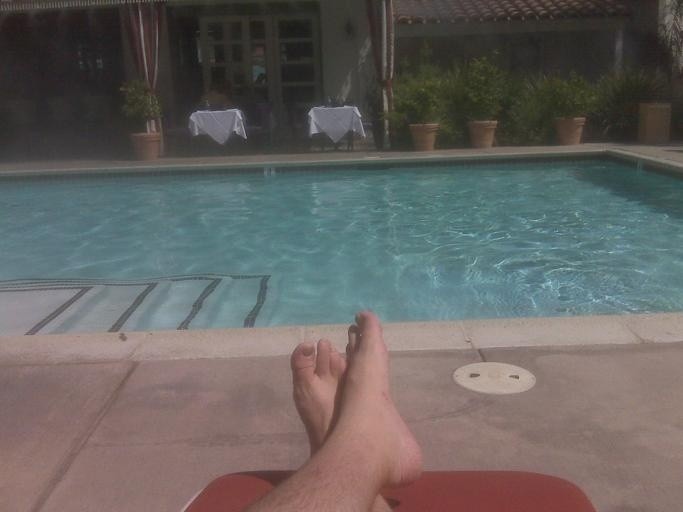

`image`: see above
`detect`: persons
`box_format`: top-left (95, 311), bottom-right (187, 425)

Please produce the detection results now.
top-left (239, 310), bottom-right (423, 512)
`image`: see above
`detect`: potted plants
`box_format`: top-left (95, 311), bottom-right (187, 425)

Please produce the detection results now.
top-left (376, 47), bottom-right (671, 151)
top-left (118, 77), bottom-right (162, 161)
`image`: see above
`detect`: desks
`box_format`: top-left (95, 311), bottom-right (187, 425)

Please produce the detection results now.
top-left (188, 109), bottom-right (248, 157)
top-left (307, 104), bottom-right (366, 152)
